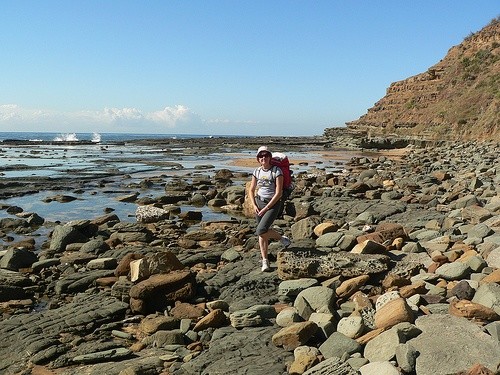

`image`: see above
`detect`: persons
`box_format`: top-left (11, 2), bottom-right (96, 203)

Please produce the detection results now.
top-left (249, 146), bottom-right (291, 272)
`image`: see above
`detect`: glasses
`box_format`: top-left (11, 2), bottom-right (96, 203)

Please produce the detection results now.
top-left (258, 153), bottom-right (270, 158)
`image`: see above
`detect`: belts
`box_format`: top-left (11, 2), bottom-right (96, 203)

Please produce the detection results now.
top-left (254, 195), bottom-right (269, 203)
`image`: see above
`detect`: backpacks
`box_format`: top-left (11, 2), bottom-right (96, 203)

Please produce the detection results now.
top-left (256, 152), bottom-right (290, 189)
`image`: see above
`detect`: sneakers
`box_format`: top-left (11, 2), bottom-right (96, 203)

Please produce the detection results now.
top-left (262, 259), bottom-right (270, 272)
top-left (280, 236), bottom-right (291, 248)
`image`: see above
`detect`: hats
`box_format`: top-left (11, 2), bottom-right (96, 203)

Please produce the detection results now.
top-left (256, 146), bottom-right (272, 156)
